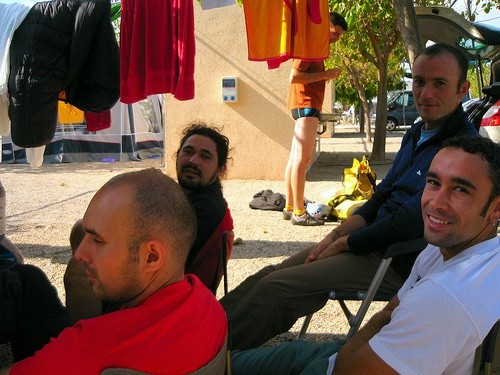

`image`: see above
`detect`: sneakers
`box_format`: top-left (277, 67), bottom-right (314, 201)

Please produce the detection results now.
top-left (282, 208), bottom-right (292, 220)
top-left (290, 212), bottom-right (324, 226)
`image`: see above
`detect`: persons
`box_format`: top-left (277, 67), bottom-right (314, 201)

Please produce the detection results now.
top-left (218, 42), bottom-right (481, 351)
top-left (0, 259), bottom-right (76, 370)
top-left (223, 135), bottom-right (491, 375)
top-left (63, 121), bottom-right (234, 322)
top-left (283, 12), bottom-right (348, 225)
top-left (8, 168), bottom-right (228, 375)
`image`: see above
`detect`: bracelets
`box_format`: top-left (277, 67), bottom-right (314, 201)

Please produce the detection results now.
top-left (333, 228), bottom-right (338, 239)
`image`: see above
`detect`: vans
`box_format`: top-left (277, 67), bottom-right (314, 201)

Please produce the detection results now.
top-left (370, 91), bottom-right (421, 131)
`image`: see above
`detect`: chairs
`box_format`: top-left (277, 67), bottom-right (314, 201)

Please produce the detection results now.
top-left (190, 230), bottom-right (428, 342)
